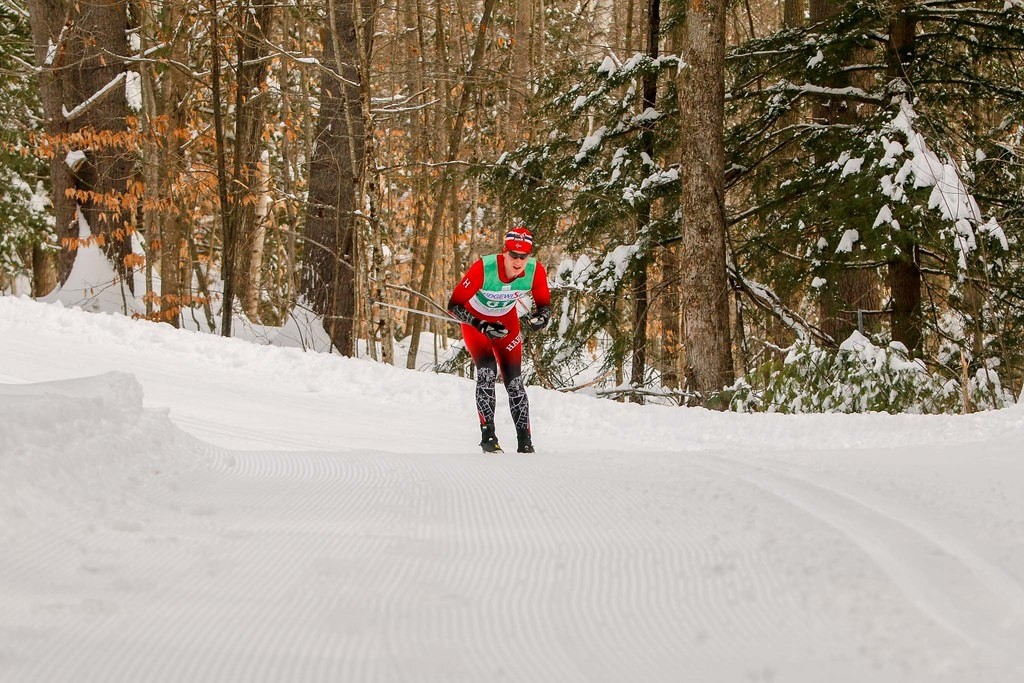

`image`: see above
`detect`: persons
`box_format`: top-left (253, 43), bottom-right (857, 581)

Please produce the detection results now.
top-left (446, 226), bottom-right (553, 456)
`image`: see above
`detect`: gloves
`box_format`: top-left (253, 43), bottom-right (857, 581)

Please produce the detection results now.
top-left (478, 319), bottom-right (511, 340)
top-left (523, 306), bottom-right (552, 331)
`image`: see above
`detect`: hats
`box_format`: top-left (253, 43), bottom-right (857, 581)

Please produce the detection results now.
top-left (505, 227), bottom-right (533, 253)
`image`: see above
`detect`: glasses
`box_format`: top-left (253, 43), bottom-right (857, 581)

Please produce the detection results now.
top-left (508, 250), bottom-right (528, 259)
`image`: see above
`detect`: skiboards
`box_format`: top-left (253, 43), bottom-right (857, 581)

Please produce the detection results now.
top-left (479, 441), bottom-right (536, 454)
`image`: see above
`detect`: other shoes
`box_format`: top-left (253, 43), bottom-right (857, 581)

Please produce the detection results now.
top-left (479, 438), bottom-right (502, 454)
top-left (517, 440), bottom-right (535, 453)
